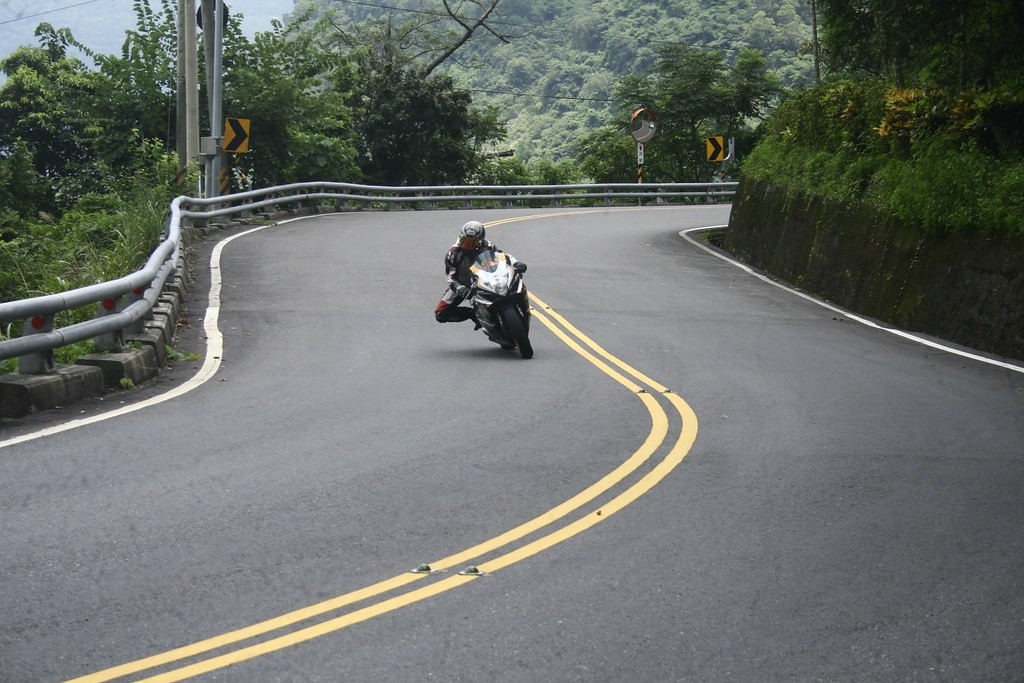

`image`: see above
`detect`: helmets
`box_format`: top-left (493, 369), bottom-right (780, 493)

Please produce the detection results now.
top-left (459, 221), bottom-right (485, 250)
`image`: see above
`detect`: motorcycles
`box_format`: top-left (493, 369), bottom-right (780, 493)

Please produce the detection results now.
top-left (450, 249), bottom-right (538, 360)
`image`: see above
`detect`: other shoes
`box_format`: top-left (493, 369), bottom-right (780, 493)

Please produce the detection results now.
top-left (467, 309), bottom-right (482, 330)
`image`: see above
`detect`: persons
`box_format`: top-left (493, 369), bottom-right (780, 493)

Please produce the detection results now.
top-left (434, 220), bottom-right (529, 332)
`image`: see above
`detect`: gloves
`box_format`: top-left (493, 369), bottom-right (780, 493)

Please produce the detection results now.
top-left (458, 287), bottom-right (473, 300)
top-left (515, 262), bottom-right (527, 272)
